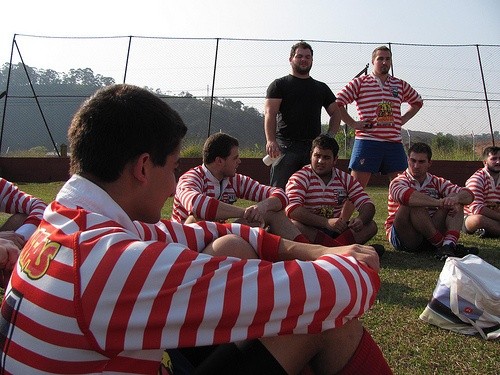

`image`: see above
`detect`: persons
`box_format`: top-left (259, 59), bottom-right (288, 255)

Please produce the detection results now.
top-left (262, 41), bottom-right (341, 192)
top-left (0, 83), bottom-right (392, 375)
top-left (285, 136), bottom-right (385, 257)
top-left (335, 45), bottom-right (423, 223)
top-left (171, 132), bottom-right (312, 255)
top-left (461, 146), bottom-right (500, 238)
top-left (0, 176), bottom-right (49, 292)
top-left (384, 141), bottom-right (479, 262)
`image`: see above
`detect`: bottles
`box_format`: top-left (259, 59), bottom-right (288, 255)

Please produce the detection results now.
top-left (262, 144), bottom-right (288, 166)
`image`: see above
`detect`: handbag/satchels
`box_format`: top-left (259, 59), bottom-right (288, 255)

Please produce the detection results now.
top-left (419, 254), bottom-right (500, 340)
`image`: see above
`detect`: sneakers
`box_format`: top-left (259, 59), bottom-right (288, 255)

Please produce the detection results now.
top-left (434, 242), bottom-right (461, 261)
top-left (456, 244), bottom-right (479, 258)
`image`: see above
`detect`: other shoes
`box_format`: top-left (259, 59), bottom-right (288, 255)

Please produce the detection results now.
top-left (369, 244), bottom-right (385, 258)
top-left (474, 228), bottom-right (486, 238)
